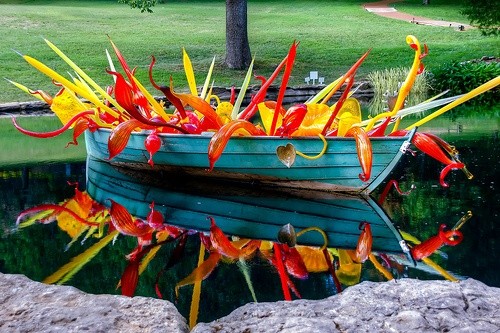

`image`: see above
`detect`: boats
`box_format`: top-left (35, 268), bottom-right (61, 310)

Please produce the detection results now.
top-left (1, 32), bottom-right (499, 196)
top-left (18, 155), bottom-right (472, 333)
